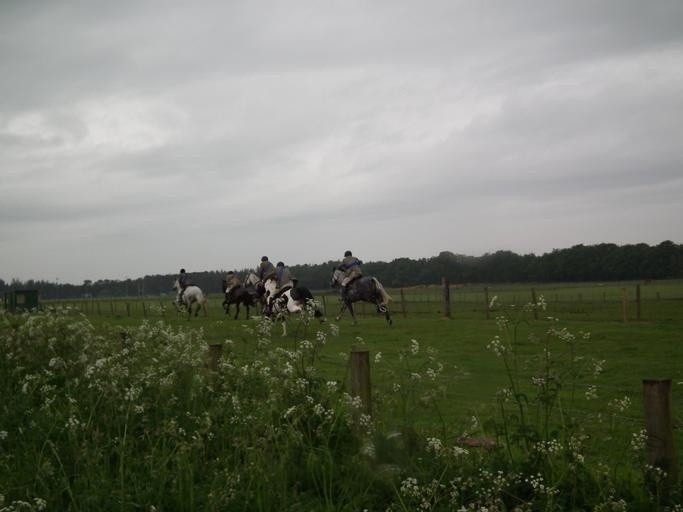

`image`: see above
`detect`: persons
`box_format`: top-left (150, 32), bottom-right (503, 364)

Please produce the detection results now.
top-left (179, 269), bottom-right (196, 304)
top-left (256, 256), bottom-right (277, 298)
top-left (225, 271), bottom-right (240, 303)
top-left (259, 261), bottom-right (294, 316)
top-left (338, 251), bottom-right (363, 301)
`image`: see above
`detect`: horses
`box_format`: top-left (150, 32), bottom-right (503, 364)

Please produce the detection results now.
top-left (222, 269), bottom-right (327, 337)
top-left (330, 265), bottom-right (393, 327)
top-left (172, 278), bottom-right (209, 321)
